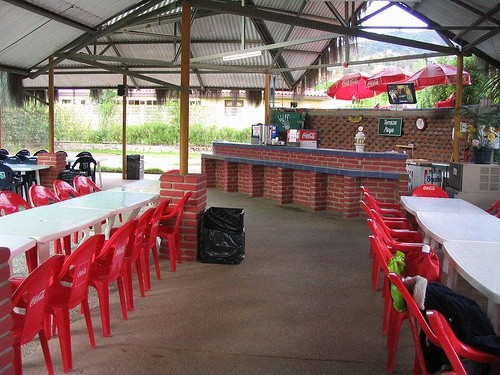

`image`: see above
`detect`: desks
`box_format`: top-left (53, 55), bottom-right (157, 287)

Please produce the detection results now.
top-left (0, 234), bottom-right (36, 279)
top-left (4, 164), bottom-right (50, 203)
top-left (64, 156), bottom-right (108, 188)
top-left (413, 212), bottom-right (500, 290)
top-left (400, 195), bottom-right (482, 213)
top-left (0, 205), bottom-right (113, 266)
top-left (108, 180), bottom-right (161, 194)
top-left (47, 190), bottom-right (159, 240)
top-left (441, 241), bottom-right (500, 340)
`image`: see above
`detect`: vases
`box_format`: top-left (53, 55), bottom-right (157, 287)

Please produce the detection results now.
top-left (473, 147), bottom-right (492, 165)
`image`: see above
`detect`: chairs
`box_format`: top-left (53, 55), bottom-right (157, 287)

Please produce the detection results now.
top-left (0, 149), bottom-right (193, 375)
top-left (359, 184), bottom-right (500, 375)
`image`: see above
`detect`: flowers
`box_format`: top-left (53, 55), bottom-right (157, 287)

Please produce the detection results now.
top-left (456, 105), bottom-right (500, 150)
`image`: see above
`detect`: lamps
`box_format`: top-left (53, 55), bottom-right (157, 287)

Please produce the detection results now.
top-left (223, 51), bottom-right (264, 62)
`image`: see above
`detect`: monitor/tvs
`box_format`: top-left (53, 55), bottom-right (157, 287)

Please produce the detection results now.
top-left (387, 83), bottom-right (417, 104)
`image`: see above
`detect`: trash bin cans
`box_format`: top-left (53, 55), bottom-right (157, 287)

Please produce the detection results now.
top-left (61, 171), bottom-right (88, 199)
top-left (127, 155), bottom-right (144, 180)
top-left (202, 206), bottom-right (245, 264)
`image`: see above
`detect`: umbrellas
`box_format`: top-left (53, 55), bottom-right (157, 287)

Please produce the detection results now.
top-left (406, 63), bottom-right (471, 108)
top-left (366, 67), bottom-right (414, 110)
top-left (327, 71), bottom-right (375, 107)
